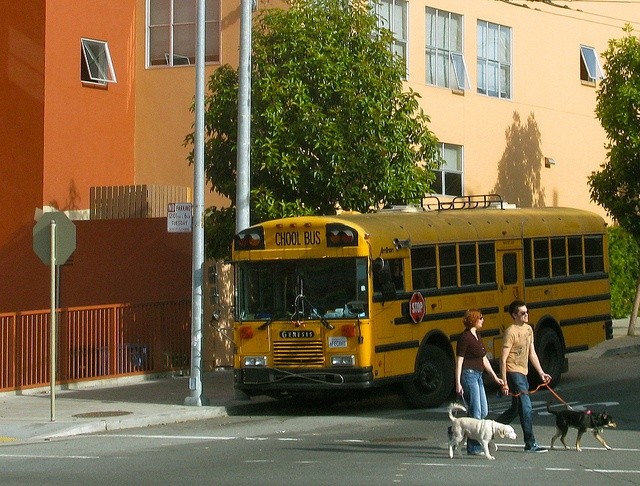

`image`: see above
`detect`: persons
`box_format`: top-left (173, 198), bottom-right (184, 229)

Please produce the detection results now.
top-left (492, 300), bottom-right (552, 453)
top-left (447, 309), bottom-right (505, 456)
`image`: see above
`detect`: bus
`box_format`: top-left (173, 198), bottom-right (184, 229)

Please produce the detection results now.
top-left (232, 194), bottom-right (612, 409)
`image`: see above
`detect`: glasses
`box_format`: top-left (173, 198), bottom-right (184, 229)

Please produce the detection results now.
top-left (480, 317), bottom-right (484, 320)
top-left (519, 311), bottom-right (529, 315)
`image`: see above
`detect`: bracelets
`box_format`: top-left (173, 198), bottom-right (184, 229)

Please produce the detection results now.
top-left (539, 373), bottom-right (545, 375)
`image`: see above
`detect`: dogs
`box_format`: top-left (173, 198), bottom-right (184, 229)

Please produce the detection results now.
top-left (448, 403), bottom-right (518, 460)
top-left (547, 400), bottom-right (617, 452)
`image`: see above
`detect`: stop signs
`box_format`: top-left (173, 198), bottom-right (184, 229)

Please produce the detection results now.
top-left (409, 292), bottom-right (425, 323)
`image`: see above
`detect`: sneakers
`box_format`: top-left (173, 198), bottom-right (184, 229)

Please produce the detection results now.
top-left (525, 443), bottom-right (549, 453)
top-left (467, 449), bottom-right (484, 456)
